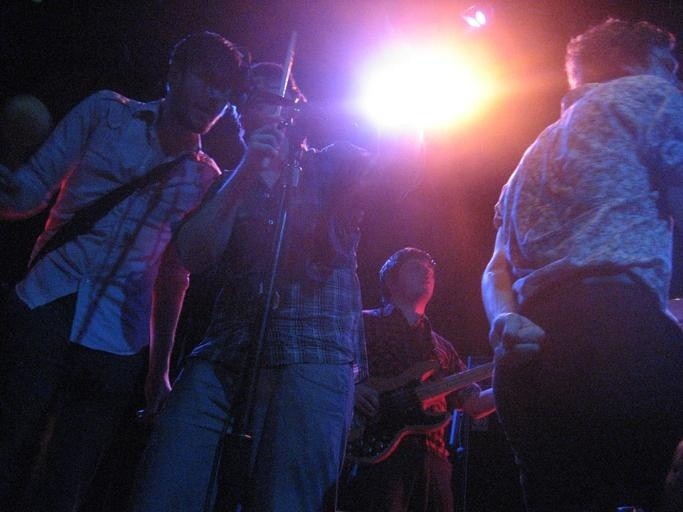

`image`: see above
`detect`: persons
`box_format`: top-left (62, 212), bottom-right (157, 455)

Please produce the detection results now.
top-left (482, 19), bottom-right (683, 511)
top-left (348, 247), bottom-right (497, 511)
top-left (138, 60), bottom-right (426, 512)
top-left (0, 32), bottom-right (245, 512)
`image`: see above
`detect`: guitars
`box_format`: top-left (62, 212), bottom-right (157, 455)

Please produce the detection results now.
top-left (347, 360), bottom-right (493, 464)
